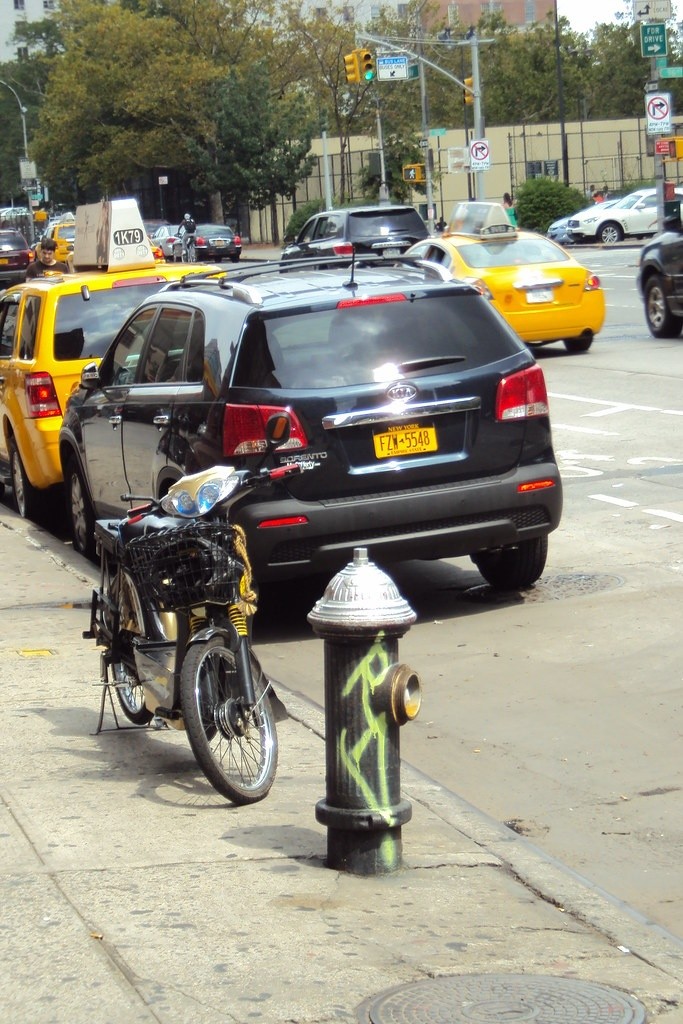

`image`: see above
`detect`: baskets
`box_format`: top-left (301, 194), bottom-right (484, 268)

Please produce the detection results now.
top-left (126, 521), bottom-right (241, 613)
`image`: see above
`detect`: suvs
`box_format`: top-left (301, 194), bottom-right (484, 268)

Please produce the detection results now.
top-left (59, 253), bottom-right (562, 632)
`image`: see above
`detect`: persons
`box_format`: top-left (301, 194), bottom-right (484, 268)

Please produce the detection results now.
top-left (603, 186), bottom-right (610, 198)
top-left (25, 237), bottom-right (69, 283)
top-left (593, 193), bottom-right (603, 202)
top-left (434, 217), bottom-right (447, 232)
top-left (587, 185), bottom-right (595, 199)
top-left (177, 213), bottom-right (196, 255)
top-left (502, 192), bottom-right (518, 227)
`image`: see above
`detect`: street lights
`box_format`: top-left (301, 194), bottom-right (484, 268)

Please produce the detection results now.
top-left (437, 25), bottom-right (475, 202)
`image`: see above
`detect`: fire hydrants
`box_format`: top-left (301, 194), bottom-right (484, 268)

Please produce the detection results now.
top-left (307, 547), bottom-right (422, 872)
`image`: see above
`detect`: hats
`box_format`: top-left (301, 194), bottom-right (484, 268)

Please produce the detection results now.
top-left (184, 213), bottom-right (191, 219)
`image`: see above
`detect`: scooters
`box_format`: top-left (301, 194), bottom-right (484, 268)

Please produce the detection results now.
top-left (82, 412), bottom-right (303, 804)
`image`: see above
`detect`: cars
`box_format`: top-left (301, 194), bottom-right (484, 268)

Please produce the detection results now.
top-left (636, 215), bottom-right (683, 339)
top-left (547, 188), bottom-right (683, 244)
top-left (0, 264), bottom-right (226, 518)
top-left (279, 205), bottom-right (430, 274)
top-left (142, 219), bottom-right (242, 263)
top-left (393, 230), bottom-right (606, 351)
top-left (0, 207), bottom-right (76, 282)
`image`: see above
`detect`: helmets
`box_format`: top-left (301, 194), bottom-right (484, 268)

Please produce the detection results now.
top-left (149, 539), bottom-right (235, 606)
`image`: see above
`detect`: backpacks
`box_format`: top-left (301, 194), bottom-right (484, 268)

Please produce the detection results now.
top-left (185, 219), bottom-right (195, 234)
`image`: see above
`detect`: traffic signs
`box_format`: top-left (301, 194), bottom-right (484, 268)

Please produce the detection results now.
top-left (375, 57), bottom-right (409, 82)
top-left (641, 80), bottom-right (658, 94)
top-left (418, 139), bottom-right (428, 148)
top-left (640, 23), bottom-right (667, 57)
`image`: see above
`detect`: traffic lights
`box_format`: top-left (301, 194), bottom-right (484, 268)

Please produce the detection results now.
top-left (463, 77), bottom-right (475, 105)
top-left (344, 49), bottom-right (373, 84)
top-left (669, 140), bottom-right (683, 159)
top-left (402, 167), bottom-right (422, 182)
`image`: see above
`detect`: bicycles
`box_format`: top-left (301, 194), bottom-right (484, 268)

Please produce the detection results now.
top-left (174, 234), bottom-right (197, 263)
